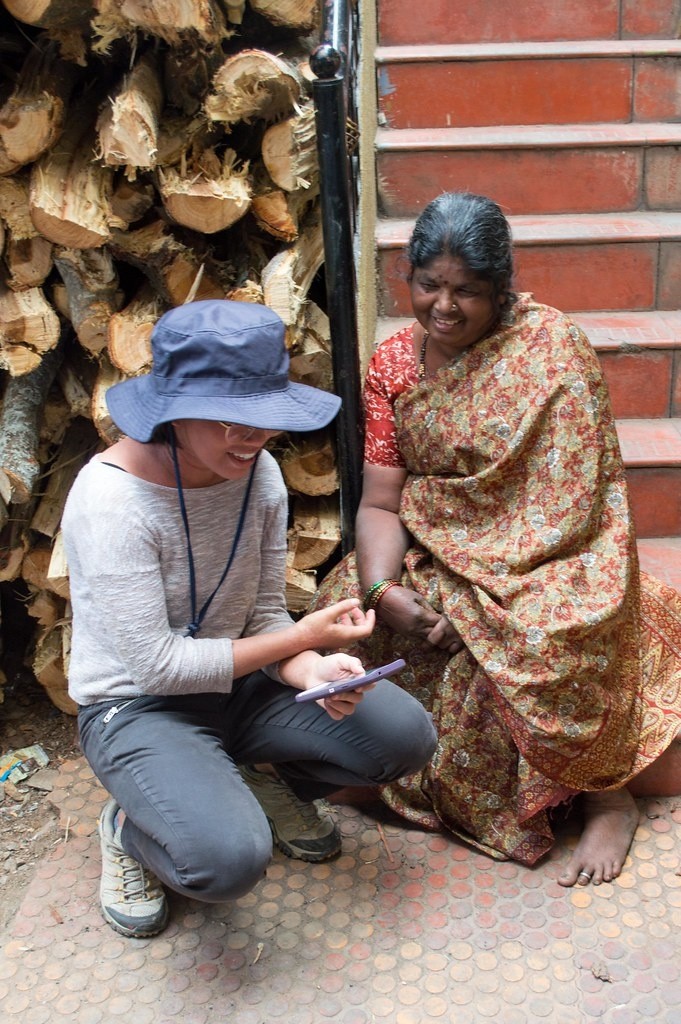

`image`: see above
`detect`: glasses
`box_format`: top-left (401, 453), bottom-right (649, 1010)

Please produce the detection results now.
top-left (215, 422), bottom-right (284, 445)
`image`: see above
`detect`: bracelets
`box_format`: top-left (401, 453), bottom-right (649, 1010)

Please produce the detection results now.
top-left (362, 578), bottom-right (404, 612)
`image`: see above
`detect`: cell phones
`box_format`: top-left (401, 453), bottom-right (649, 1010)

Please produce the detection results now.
top-left (295, 659), bottom-right (407, 703)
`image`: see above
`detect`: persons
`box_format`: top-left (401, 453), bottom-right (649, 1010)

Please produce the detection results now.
top-left (64, 297), bottom-right (439, 938)
top-left (306, 192), bottom-right (642, 889)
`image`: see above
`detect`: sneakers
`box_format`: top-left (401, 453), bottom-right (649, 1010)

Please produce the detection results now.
top-left (100, 799), bottom-right (168, 937)
top-left (237, 764), bottom-right (342, 864)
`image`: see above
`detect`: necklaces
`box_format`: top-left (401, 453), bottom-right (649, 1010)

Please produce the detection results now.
top-left (164, 426), bottom-right (262, 638)
top-left (419, 328), bottom-right (431, 382)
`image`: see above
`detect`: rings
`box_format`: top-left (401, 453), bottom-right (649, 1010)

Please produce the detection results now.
top-left (579, 872), bottom-right (591, 879)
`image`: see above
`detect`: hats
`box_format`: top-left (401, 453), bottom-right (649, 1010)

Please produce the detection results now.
top-left (105, 299), bottom-right (341, 442)
